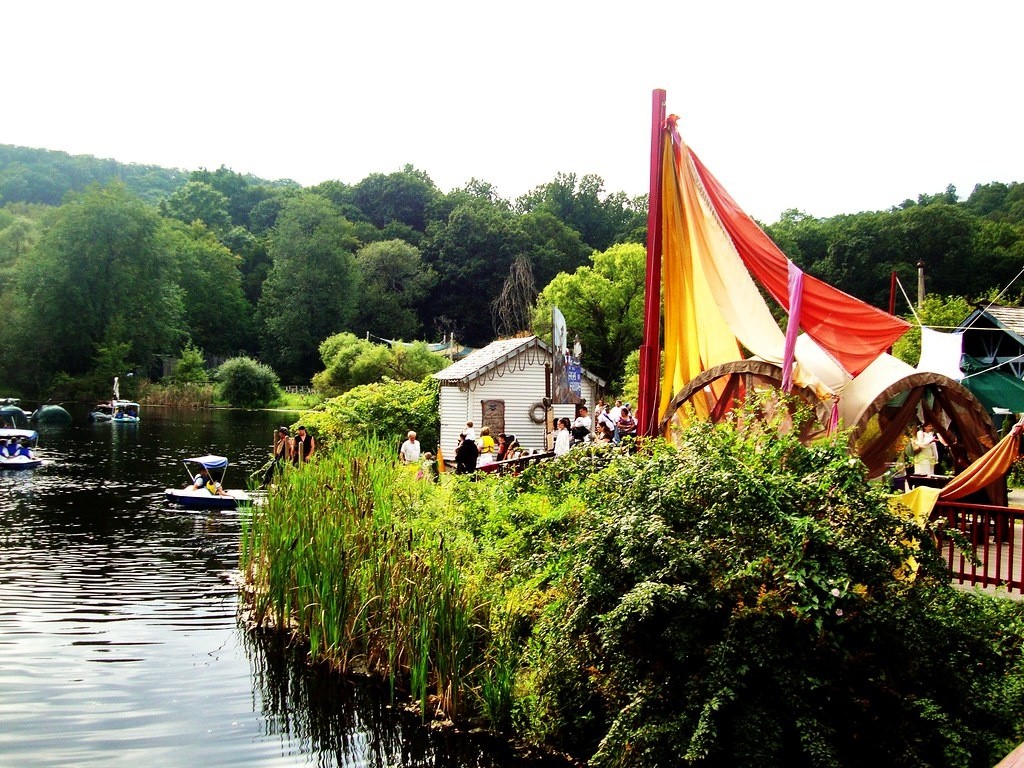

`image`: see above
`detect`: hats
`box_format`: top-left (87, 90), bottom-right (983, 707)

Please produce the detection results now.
top-left (298, 426), bottom-right (306, 430)
top-left (278, 427), bottom-right (289, 435)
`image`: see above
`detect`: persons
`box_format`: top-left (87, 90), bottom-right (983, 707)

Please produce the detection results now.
top-left (454, 421), bottom-right (530, 482)
top-left (400, 431), bottom-right (433, 481)
top-left (114, 407), bottom-right (136, 419)
top-left (256, 426), bottom-right (314, 490)
top-left (552, 398), bottom-right (637, 462)
top-left (913, 423), bottom-right (939, 478)
top-left (0, 436), bottom-right (35, 460)
top-left (192, 466), bottom-right (236, 499)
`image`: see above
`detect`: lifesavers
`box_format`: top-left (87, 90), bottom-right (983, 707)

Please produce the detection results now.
top-left (529, 402), bottom-right (545, 422)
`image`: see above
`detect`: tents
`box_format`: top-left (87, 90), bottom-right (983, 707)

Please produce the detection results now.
top-left (366, 331), bottom-right (479, 363)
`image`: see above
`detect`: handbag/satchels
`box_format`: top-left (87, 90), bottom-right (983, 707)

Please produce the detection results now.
top-left (913, 431), bottom-right (926, 453)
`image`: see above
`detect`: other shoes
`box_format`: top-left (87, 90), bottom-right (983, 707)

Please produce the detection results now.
top-left (258, 485), bottom-right (268, 490)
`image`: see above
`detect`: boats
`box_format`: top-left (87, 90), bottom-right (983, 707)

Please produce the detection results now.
top-left (111, 399), bottom-right (141, 422)
top-left (89, 400), bottom-right (113, 422)
top-left (164, 454), bottom-right (255, 510)
top-left (0, 428), bottom-right (41, 470)
